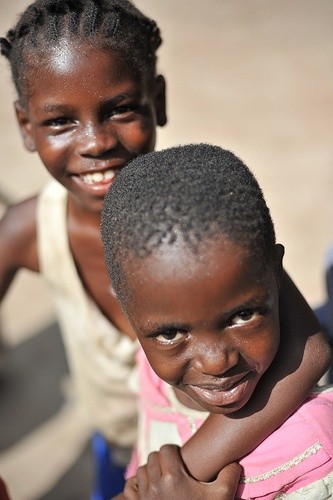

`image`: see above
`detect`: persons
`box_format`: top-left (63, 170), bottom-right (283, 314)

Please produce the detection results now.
top-left (0, 1), bottom-right (331, 499)
top-left (100, 144), bottom-right (329, 500)
